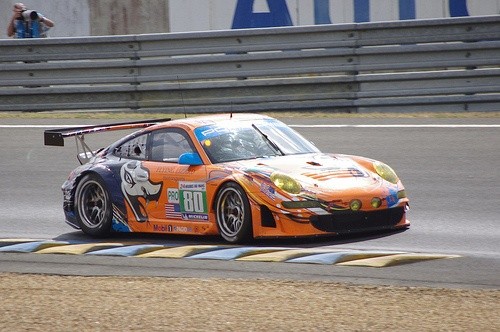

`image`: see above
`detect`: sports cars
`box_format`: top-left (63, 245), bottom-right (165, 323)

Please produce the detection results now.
top-left (44, 112), bottom-right (411, 242)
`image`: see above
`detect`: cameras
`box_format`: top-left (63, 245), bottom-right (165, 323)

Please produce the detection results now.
top-left (17, 10), bottom-right (38, 21)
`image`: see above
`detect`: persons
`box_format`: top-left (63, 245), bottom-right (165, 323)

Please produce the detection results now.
top-left (6, 2), bottom-right (55, 39)
